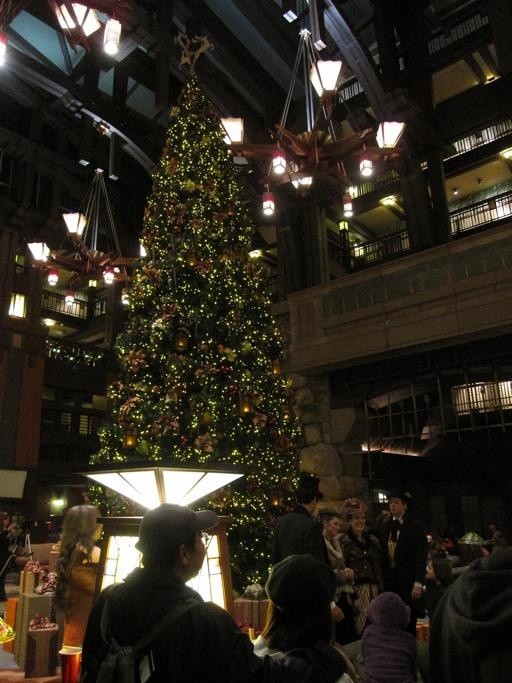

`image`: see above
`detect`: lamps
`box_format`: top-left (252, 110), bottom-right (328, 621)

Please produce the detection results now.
top-left (78, 461), bottom-right (250, 610)
top-left (48, 0), bottom-right (132, 57)
top-left (216, 0), bottom-right (408, 218)
top-left (9, 168), bottom-right (129, 327)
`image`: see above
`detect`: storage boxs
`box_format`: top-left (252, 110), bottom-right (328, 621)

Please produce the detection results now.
top-left (234, 597), bottom-right (270, 634)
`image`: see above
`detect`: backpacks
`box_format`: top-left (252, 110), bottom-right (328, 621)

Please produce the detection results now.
top-left (81, 582), bottom-right (201, 683)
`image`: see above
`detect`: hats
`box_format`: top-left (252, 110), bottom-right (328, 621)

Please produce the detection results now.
top-left (264, 553), bottom-right (338, 612)
top-left (295, 477), bottom-right (324, 498)
top-left (139, 503), bottom-right (218, 552)
top-left (341, 499), bottom-right (366, 514)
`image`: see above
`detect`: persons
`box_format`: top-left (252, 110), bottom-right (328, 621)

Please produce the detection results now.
top-left (269, 477), bottom-right (330, 566)
top-left (78, 503), bottom-right (361, 682)
top-left (51, 505), bottom-right (103, 681)
top-left (250, 554), bottom-right (352, 682)
top-left (338, 498), bottom-right (380, 639)
top-left (378, 491), bottom-right (426, 639)
top-left (0, 511), bottom-right (68, 605)
top-left (318, 508), bottom-right (358, 646)
top-left (360, 591), bottom-right (418, 682)
top-left (428, 550), bottom-right (511, 682)
top-left (374, 511), bottom-right (512, 578)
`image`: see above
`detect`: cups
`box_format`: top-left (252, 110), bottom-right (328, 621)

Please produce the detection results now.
top-left (58, 647), bottom-right (81, 683)
